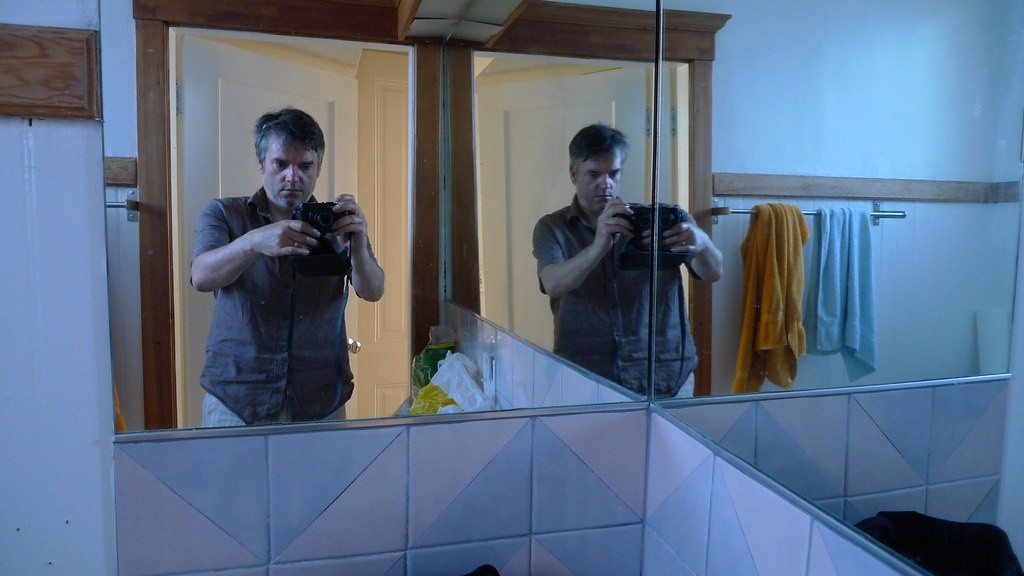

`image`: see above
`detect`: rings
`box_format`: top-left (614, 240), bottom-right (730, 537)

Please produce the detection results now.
top-left (294, 242), bottom-right (298, 247)
top-left (682, 241), bottom-right (686, 246)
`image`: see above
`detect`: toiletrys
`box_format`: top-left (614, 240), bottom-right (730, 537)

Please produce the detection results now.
top-left (438, 350), bottom-right (454, 371)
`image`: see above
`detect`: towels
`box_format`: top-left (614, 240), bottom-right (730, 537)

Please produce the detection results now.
top-left (732, 203), bottom-right (807, 395)
top-left (808, 208), bottom-right (877, 383)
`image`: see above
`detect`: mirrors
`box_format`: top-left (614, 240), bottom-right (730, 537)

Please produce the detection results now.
top-left (98, 0), bottom-right (1024, 576)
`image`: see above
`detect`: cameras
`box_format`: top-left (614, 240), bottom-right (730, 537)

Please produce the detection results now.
top-left (618, 202), bottom-right (682, 230)
top-left (291, 202), bottom-right (352, 234)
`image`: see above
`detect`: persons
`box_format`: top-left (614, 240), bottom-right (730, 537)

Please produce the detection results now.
top-left (533, 124), bottom-right (651, 396)
top-left (190, 108), bottom-right (385, 427)
top-left (655, 202), bottom-right (723, 398)
